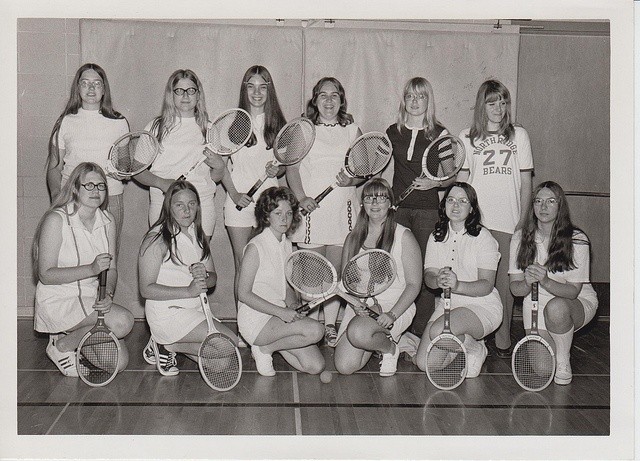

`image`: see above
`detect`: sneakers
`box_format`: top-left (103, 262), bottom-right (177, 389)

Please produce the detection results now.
top-left (554, 358), bottom-right (573, 385)
top-left (251, 344), bottom-right (277, 377)
top-left (379, 341), bottom-right (400, 377)
top-left (324, 324), bottom-right (338, 348)
top-left (46, 335), bottom-right (59, 355)
top-left (237, 332), bottom-right (249, 348)
top-left (46, 340), bottom-right (90, 377)
top-left (461, 340), bottom-right (489, 378)
top-left (150, 336), bottom-right (179, 376)
top-left (142, 335), bottom-right (157, 365)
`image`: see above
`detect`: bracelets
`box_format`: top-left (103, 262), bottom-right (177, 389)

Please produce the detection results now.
top-left (387, 311), bottom-right (396, 321)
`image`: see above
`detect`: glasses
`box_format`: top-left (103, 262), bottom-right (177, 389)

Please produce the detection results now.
top-left (404, 93), bottom-right (428, 101)
top-left (172, 87), bottom-right (198, 96)
top-left (533, 198), bottom-right (560, 206)
top-left (445, 196), bottom-right (471, 206)
top-left (74, 182), bottom-right (106, 191)
top-left (362, 195), bottom-right (389, 203)
top-left (78, 80), bottom-right (104, 88)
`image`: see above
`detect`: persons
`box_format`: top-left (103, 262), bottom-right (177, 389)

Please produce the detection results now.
top-left (414, 181), bottom-right (504, 379)
top-left (45, 62), bottom-right (134, 302)
top-left (285, 76), bottom-right (370, 349)
top-left (236, 186), bottom-right (326, 377)
top-left (370, 76), bottom-right (454, 363)
top-left (131, 67), bottom-right (223, 376)
top-left (223, 64), bottom-right (285, 349)
top-left (454, 79), bottom-right (534, 360)
top-left (508, 180), bottom-right (601, 386)
top-left (33, 162), bottom-right (135, 378)
top-left (137, 180), bottom-right (238, 377)
top-left (333, 180), bottom-right (423, 377)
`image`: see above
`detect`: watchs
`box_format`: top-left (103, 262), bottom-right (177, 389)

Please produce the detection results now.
top-left (437, 180), bottom-right (443, 188)
top-left (204, 270), bottom-right (210, 279)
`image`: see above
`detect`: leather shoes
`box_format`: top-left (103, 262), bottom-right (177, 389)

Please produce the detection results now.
top-left (494, 342), bottom-right (512, 358)
